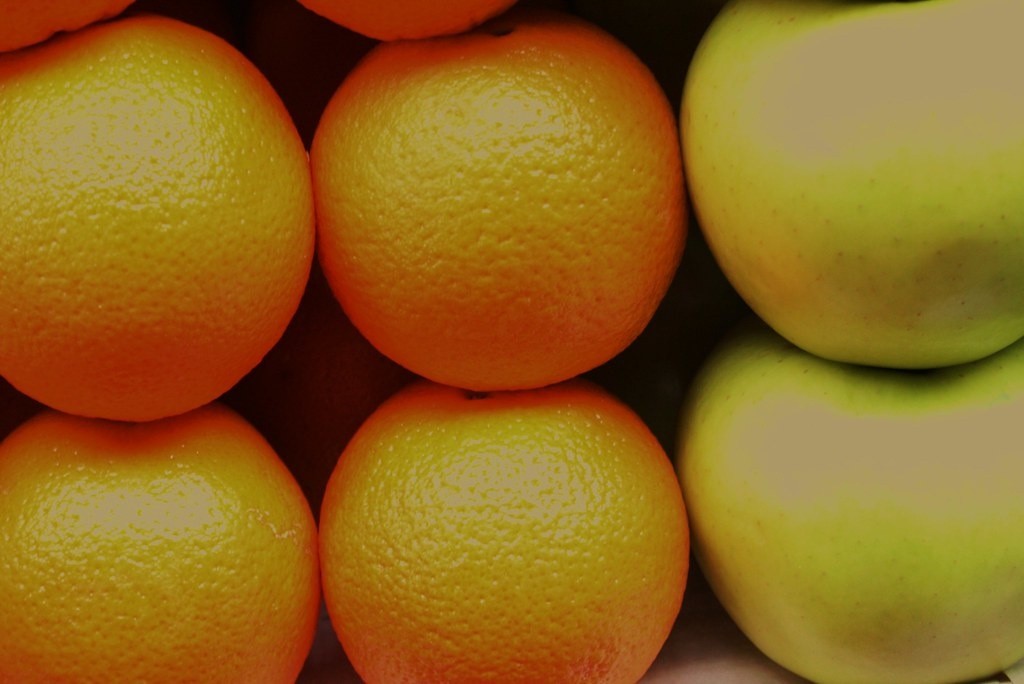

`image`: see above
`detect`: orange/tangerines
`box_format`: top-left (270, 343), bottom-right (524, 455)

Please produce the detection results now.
top-left (0, 1), bottom-right (691, 684)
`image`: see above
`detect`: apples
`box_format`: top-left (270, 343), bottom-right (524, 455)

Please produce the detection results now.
top-left (677, 0), bottom-right (1024, 684)
